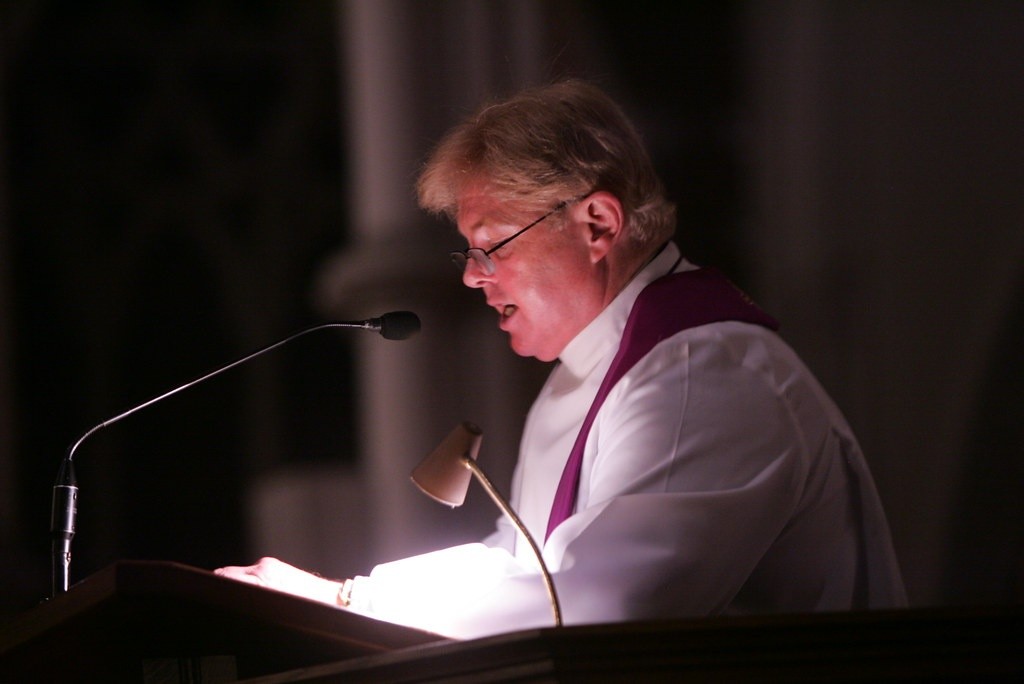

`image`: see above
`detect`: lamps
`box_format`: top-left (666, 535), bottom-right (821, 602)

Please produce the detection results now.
top-left (411, 423), bottom-right (563, 627)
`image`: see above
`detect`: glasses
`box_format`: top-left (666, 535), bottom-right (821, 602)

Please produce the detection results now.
top-left (450, 195), bottom-right (588, 277)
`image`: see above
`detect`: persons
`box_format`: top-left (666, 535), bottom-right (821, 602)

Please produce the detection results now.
top-left (212, 83), bottom-right (906, 641)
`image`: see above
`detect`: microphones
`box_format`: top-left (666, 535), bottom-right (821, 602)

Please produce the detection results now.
top-left (51, 310), bottom-right (422, 596)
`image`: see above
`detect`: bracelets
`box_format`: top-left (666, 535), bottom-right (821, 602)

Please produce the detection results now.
top-left (336, 578), bottom-right (353, 608)
